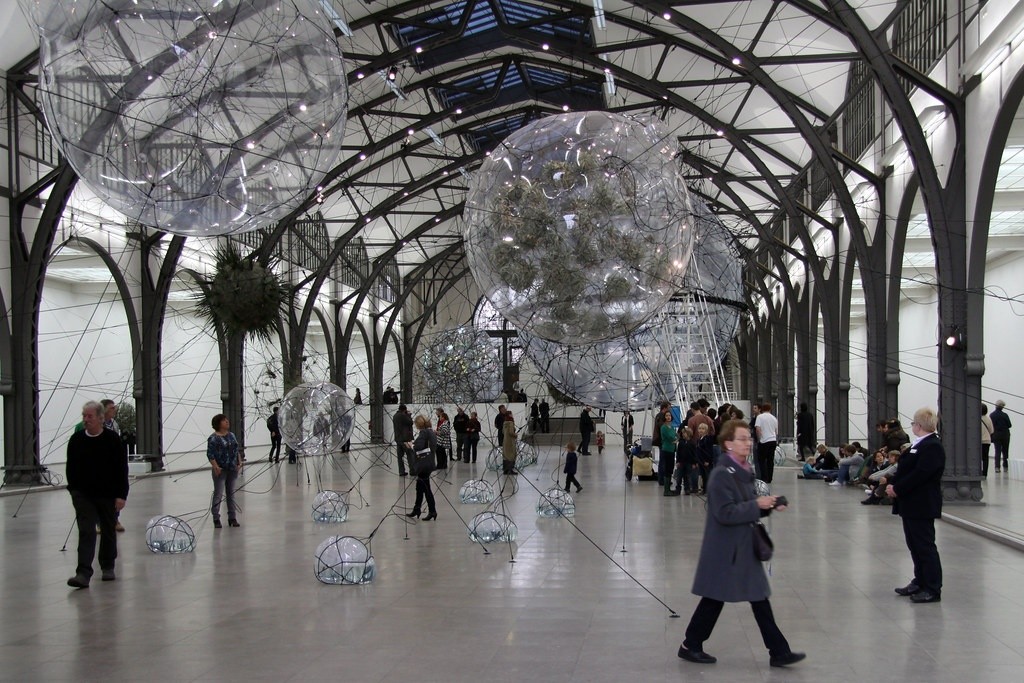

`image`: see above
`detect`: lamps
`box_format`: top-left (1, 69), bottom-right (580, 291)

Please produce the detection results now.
top-left (946, 335), bottom-right (966, 351)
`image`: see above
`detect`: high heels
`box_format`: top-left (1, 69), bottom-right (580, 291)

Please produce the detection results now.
top-left (406, 508), bottom-right (422, 519)
top-left (228, 518), bottom-right (240, 527)
top-left (422, 511), bottom-right (438, 521)
top-left (213, 519), bottom-right (223, 528)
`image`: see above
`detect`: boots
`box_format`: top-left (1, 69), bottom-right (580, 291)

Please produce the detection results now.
top-left (663, 478), bottom-right (676, 496)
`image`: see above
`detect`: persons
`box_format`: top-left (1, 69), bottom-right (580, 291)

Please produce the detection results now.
top-left (891, 407), bottom-right (946, 603)
top-left (796, 403), bottom-right (815, 462)
top-left (383, 387), bottom-right (398, 404)
top-left (531, 399), bottom-right (551, 434)
top-left (340, 438), bottom-right (351, 452)
top-left (495, 405), bottom-right (518, 475)
top-left (67, 400), bottom-right (130, 589)
top-left (620, 411), bottom-right (635, 446)
top-left (563, 443), bottom-right (583, 493)
top-left (354, 388), bottom-right (362, 404)
top-left (802, 418), bottom-right (910, 506)
top-left (576, 406), bottom-right (594, 456)
top-left (989, 400), bottom-right (1012, 473)
top-left (651, 402), bottom-right (777, 497)
top-left (207, 414), bottom-right (240, 528)
top-left (981, 403), bottom-right (994, 479)
top-left (267, 407), bottom-right (296, 463)
top-left (393, 404), bottom-right (481, 522)
top-left (678, 420), bottom-right (806, 668)
top-left (596, 430), bottom-right (604, 454)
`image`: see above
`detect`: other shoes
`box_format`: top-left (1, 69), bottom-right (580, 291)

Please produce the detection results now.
top-left (503, 469), bottom-right (518, 475)
top-left (860, 493), bottom-right (880, 505)
top-left (399, 471), bottom-right (408, 476)
top-left (576, 486), bottom-right (583, 493)
top-left (677, 643), bottom-right (717, 663)
top-left (564, 488), bottom-right (570, 493)
top-left (828, 480), bottom-right (842, 486)
top-left (769, 651), bottom-right (806, 667)
top-left (1004, 467), bottom-right (1009, 472)
top-left (67, 570), bottom-right (91, 588)
top-left (995, 467), bottom-right (1001, 473)
top-left (95, 525), bottom-right (102, 534)
top-left (115, 521), bottom-right (125, 532)
top-left (798, 458), bottom-right (805, 461)
top-left (102, 568), bottom-right (116, 581)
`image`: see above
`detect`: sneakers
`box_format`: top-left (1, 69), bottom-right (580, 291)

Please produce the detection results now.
top-left (894, 582), bottom-right (921, 596)
top-left (910, 589), bottom-right (942, 603)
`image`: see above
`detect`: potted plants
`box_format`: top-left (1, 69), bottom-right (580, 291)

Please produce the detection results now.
top-left (115, 401), bottom-right (137, 458)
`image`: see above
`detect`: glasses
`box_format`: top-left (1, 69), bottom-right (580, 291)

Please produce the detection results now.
top-left (734, 437), bottom-right (754, 444)
top-left (911, 422), bottom-right (921, 428)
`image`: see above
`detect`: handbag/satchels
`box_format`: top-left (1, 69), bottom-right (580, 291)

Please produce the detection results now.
top-left (752, 518), bottom-right (774, 562)
top-left (405, 429), bottom-right (437, 476)
